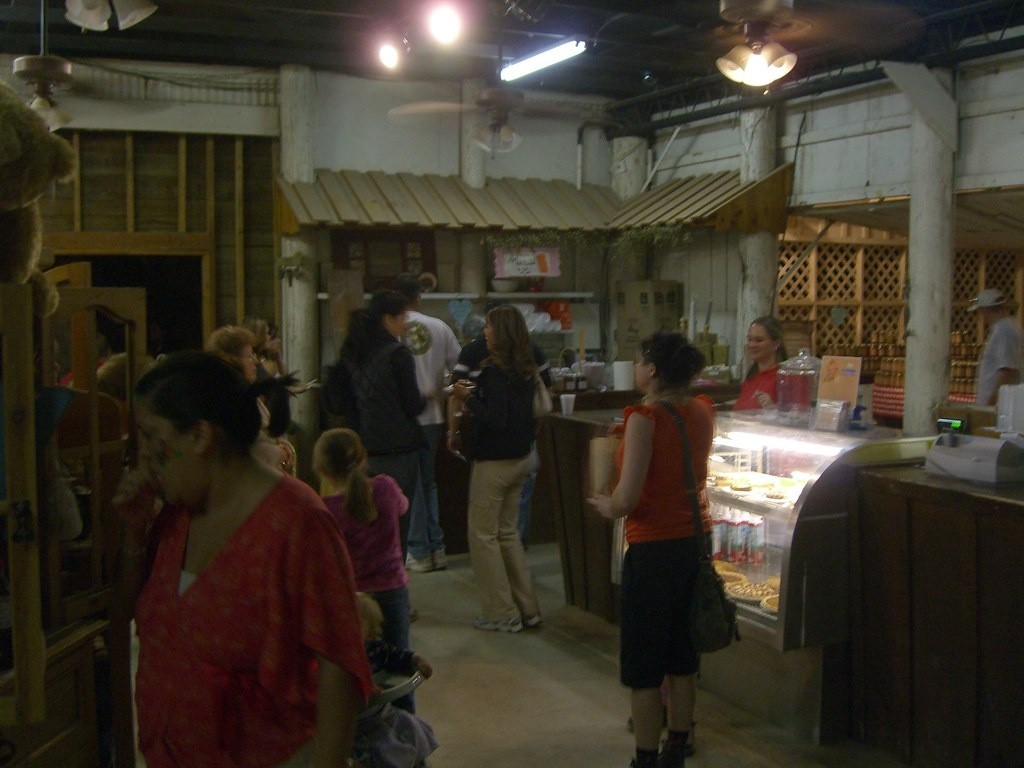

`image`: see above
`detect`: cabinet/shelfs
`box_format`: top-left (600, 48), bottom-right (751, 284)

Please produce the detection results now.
top-left (608, 412), bottom-right (878, 745)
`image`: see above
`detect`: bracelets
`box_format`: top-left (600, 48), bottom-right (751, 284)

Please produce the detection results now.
top-left (122, 545), bottom-right (146, 560)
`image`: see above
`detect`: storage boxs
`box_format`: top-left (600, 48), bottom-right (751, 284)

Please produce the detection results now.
top-left (614, 278), bottom-right (681, 367)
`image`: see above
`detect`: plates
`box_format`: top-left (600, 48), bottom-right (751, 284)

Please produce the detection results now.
top-left (758, 493), bottom-right (794, 505)
top-left (712, 559), bottom-right (780, 613)
top-left (722, 485), bottom-right (762, 497)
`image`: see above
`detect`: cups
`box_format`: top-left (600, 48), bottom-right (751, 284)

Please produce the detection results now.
top-left (584, 362), bottom-right (606, 388)
top-left (560, 394), bottom-right (576, 416)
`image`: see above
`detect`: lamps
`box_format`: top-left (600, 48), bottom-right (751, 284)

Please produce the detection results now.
top-left (64, 0), bottom-right (158, 33)
top-left (716, 0), bottom-right (798, 87)
top-left (495, 31), bottom-right (591, 82)
top-left (12, 0), bottom-right (75, 133)
top-left (470, 40), bottom-right (529, 162)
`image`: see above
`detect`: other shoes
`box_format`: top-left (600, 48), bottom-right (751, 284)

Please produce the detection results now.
top-left (626, 706), bottom-right (669, 733)
top-left (405, 547), bottom-right (447, 571)
top-left (628, 741), bottom-right (685, 768)
top-left (475, 612), bottom-right (541, 632)
top-left (684, 722), bottom-right (698, 756)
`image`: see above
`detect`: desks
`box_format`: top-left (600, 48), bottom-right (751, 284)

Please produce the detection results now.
top-left (846, 452), bottom-right (1024, 768)
top-left (543, 409), bottom-right (628, 626)
top-left (0, 260), bottom-right (146, 768)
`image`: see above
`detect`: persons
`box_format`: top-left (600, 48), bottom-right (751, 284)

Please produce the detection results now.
top-left (333, 293), bottom-right (431, 623)
top-left (584, 329), bottom-right (718, 768)
top-left (391, 272), bottom-right (464, 574)
top-left (209, 315), bottom-right (435, 710)
top-left (449, 298), bottom-right (556, 633)
top-left (977, 288), bottom-right (1024, 406)
top-left (109, 347), bottom-right (371, 768)
top-left (731, 315), bottom-right (811, 425)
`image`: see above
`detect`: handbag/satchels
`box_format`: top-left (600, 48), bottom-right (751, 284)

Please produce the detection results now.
top-left (695, 562), bottom-right (741, 652)
top-left (534, 373), bottom-right (552, 418)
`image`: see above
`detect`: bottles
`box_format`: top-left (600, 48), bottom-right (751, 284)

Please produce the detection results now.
top-left (553, 372), bottom-right (588, 393)
top-left (777, 348), bottom-right (822, 430)
top-left (613, 361), bottom-right (634, 391)
